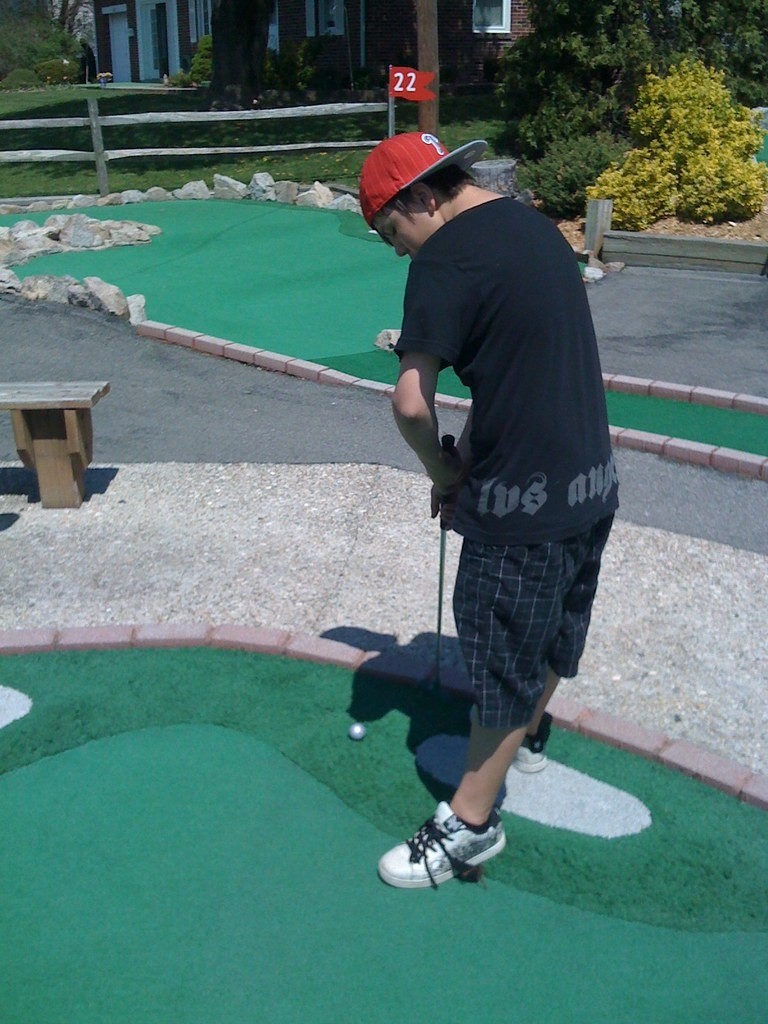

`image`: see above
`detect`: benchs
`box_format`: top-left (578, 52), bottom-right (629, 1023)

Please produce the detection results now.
top-left (1, 380), bottom-right (111, 510)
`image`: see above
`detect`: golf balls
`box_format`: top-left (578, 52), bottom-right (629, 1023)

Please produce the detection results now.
top-left (348, 722), bottom-right (367, 741)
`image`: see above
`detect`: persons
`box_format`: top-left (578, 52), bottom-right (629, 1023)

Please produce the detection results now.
top-left (359, 130), bottom-right (620, 889)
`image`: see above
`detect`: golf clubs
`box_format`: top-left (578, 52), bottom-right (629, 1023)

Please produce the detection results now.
top-left (419, 432), bottom-right (457, 702)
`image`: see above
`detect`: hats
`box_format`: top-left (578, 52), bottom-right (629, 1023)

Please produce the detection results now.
top-left (360, 132), bottom-right (488, 229)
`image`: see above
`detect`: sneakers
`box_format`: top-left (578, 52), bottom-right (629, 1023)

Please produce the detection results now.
top-left (512, 712), bottom-right (553, 773)
top-left (379, 800), bottom-right (506, 890)
